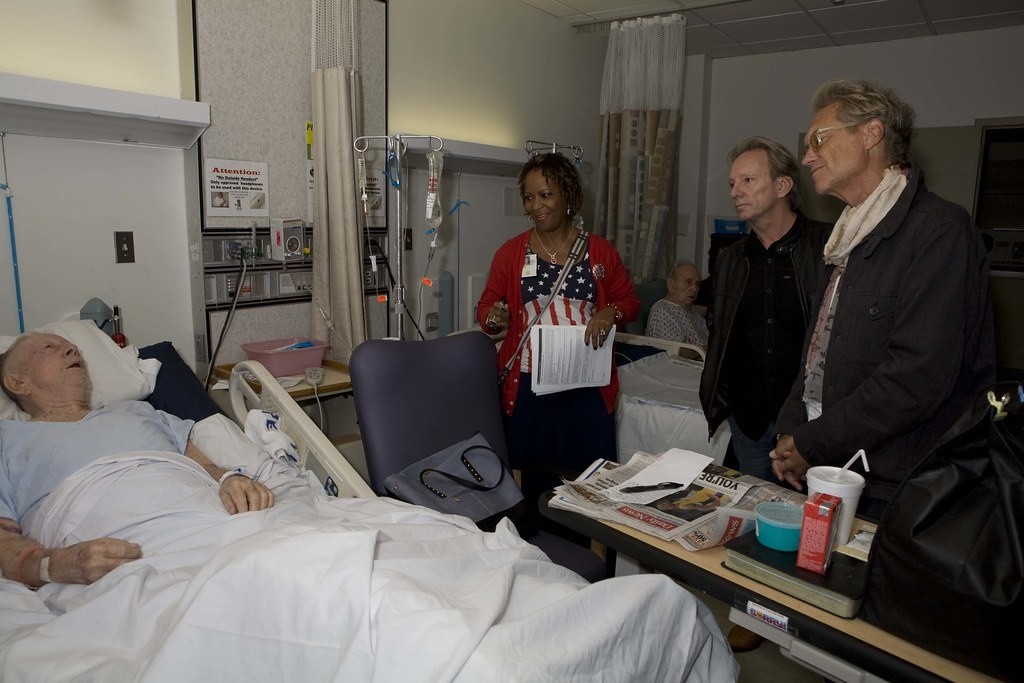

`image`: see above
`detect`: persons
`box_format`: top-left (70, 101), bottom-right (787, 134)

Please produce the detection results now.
top-left (0, 333), bottom-right (275, 585)
top-left (646, 260), bottom-right (709, 361)
top-left (477, 154), bottom-right (640, 543)
top-left (699, 137), bottom-right (828, 653)
top-left (657, 489), bottom-right (729, 511)
top-left (770, 83), bottom-right (997, 519)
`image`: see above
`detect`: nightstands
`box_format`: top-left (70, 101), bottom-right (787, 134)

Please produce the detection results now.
top-left (205, 360), bottom-right (362, 449)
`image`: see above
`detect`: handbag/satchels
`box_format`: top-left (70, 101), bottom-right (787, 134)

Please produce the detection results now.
top-left (381, 431), bottom-right (526, 525)
top-left (860, 380), bottom-right (1024, 683)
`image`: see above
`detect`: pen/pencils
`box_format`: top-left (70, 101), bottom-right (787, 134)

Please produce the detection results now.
top-left (619, 481), bottom-right (684, 493)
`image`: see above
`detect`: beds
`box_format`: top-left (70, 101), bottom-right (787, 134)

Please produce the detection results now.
top-left (613, 341), bottom-right (733, 465)
top-left (0, 341), bottom-right (376, 572)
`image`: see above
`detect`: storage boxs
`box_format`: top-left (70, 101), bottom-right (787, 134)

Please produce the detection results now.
top-left (241, 337), bottom-right (330, 377)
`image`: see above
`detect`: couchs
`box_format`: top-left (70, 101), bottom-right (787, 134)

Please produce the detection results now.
top-left (614, 277), bottom-right (707, 369)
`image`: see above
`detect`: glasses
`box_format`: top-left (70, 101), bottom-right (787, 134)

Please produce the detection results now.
top-left (802, 119), bottom-right (870, 155)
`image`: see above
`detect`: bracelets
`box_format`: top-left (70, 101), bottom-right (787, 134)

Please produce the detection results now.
top-left (219, 471), bottom-right (251, 487)
top-left (606, 304), bottom-right (620, 321)
top-left (486, 317), bottom-right (498, 330)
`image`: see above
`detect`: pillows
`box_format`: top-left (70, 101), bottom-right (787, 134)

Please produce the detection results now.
top-left (0, 317), bottom-right (163, 423)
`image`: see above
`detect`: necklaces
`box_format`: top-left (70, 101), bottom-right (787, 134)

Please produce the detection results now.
top-left (534, 223), bottom-right (574, 264)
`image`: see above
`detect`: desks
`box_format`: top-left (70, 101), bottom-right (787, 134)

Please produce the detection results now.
top-left (537, 480), bottom-right (1023, 681)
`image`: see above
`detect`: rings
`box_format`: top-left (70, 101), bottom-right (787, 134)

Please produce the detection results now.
top-left (489, 315), bottom-right (496, 325)
top-left (600, 330), bottom-right (605, 336)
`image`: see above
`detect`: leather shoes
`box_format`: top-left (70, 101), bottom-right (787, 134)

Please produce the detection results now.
top-left (727, 625), bottom-right (763, 652)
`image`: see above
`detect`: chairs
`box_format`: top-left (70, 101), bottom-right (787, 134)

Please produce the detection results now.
top-left (346, 331), bottom-right (605, 585)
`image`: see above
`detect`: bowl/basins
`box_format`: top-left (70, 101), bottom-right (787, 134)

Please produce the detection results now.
top-left (754, 502), bottom-right (806, 552)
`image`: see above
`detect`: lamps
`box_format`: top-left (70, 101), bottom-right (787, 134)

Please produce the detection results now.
top-left (390, 132), bottom-right (530, 179)
top-left (0, 72), bottom-right (212, 151)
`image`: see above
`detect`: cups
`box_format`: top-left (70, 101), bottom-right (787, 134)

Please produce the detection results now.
top-left (806, 465), bottom-right (866, 551)
